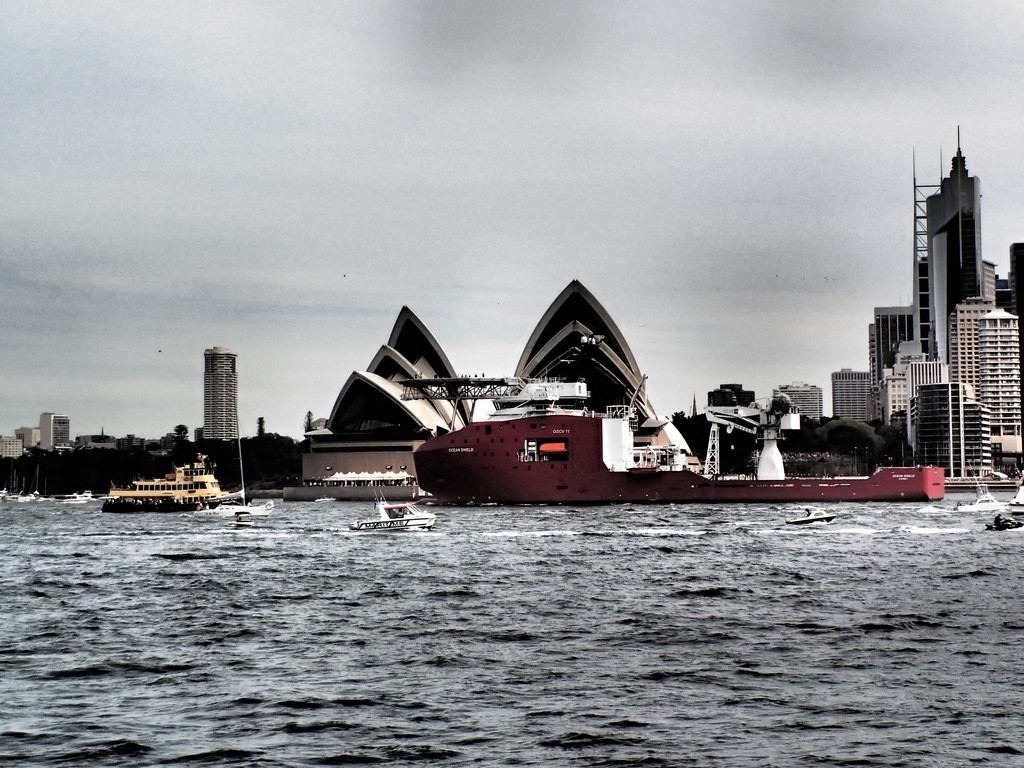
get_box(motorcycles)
[985,521,1024,532]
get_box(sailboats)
[0,466,51,503]
[193,402,274,518]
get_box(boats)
[955,459,1007,511]
[55,493,93,503]
[282,469,428,502]
[315,496,336,503]
[232,510,254,526]
[1008,465,1024,515]
[349,491,436,531]
[412,367,945,503]
[785,508,837,524]
[102,452,227,512]
